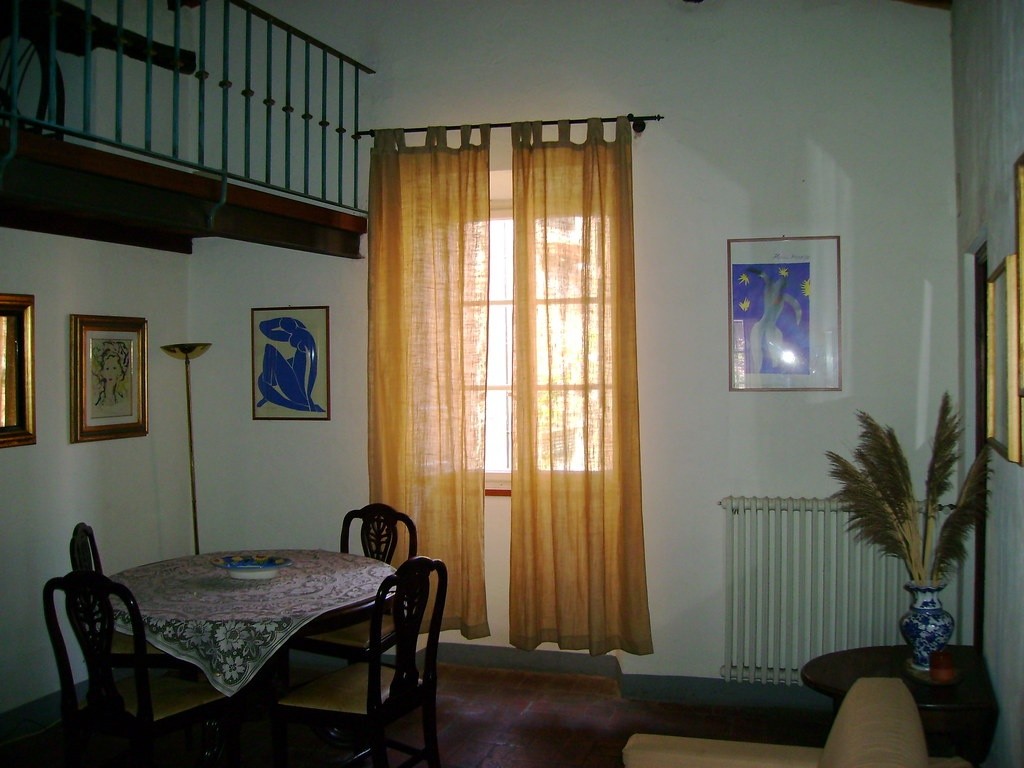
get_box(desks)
[85,550,400,700]
[801,644,999,757]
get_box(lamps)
[161,342,212,556]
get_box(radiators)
[717,495,958,688]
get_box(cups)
[930,649,954,686]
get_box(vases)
[899,583,955,670]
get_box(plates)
[208,554,294,580]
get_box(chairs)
[43,523,243,768]
[270,504,447,768]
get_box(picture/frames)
[0,293,36,449]
[727,236,842,392]
[70,314,149,444]
[985,255,1021,465]
[250,306,331,421]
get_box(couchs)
[623,673,974,768]
